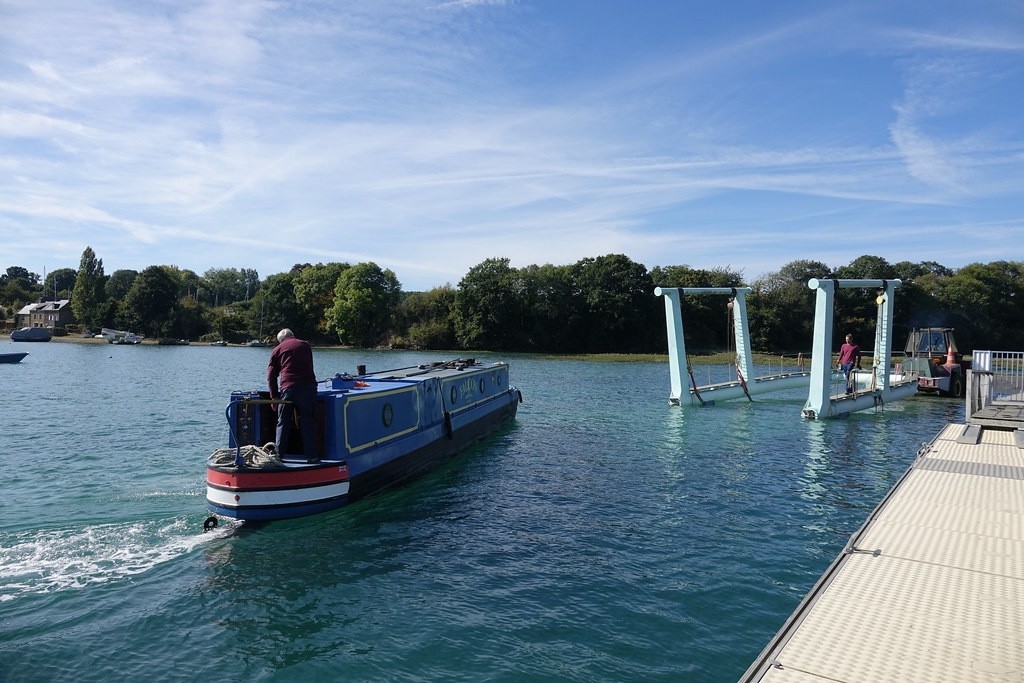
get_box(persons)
[267,328,323,463]
[837,334,862,395]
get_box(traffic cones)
[946,343,958,365]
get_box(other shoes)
[308,458,320,464]
[849,389,855,393]
[844,392,848,395]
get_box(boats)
[204,356,522,524]
[0,352,29,363]
[246,339,268,347]
[10,326,52,342]
[208,340,228,346]
